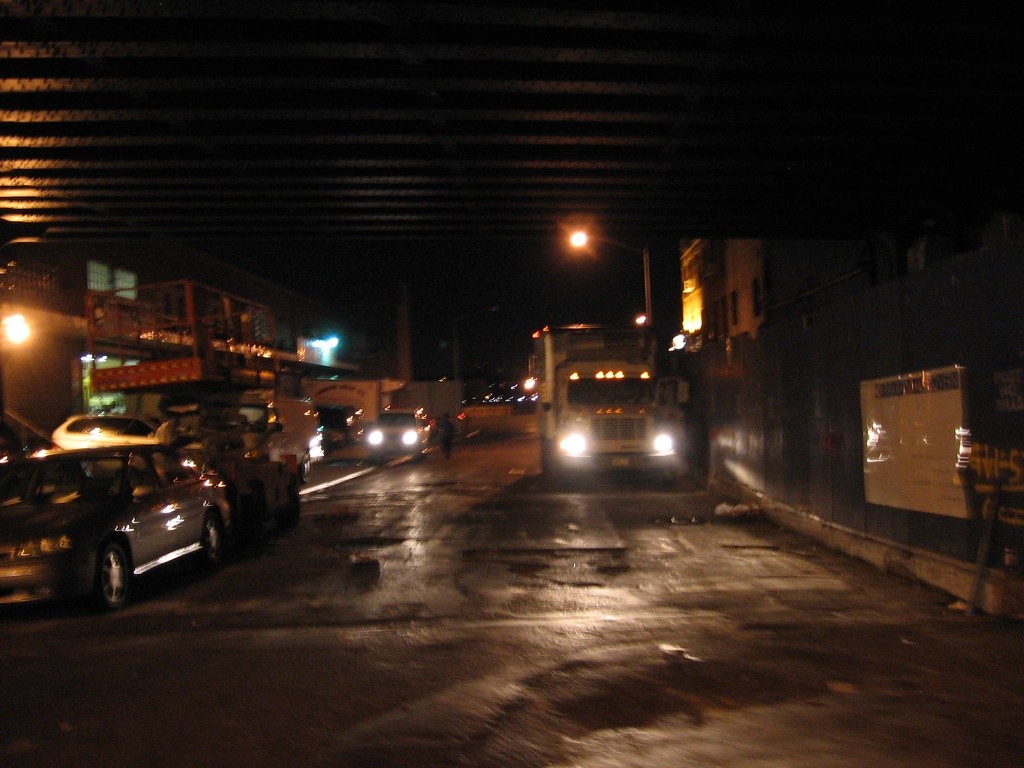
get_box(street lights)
[568,228,656,324]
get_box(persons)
[438,413,456,458]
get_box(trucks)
[528,324,693,488]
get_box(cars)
[0,411,178,453]
[364,407,436,459]
[0,443,233,614]
